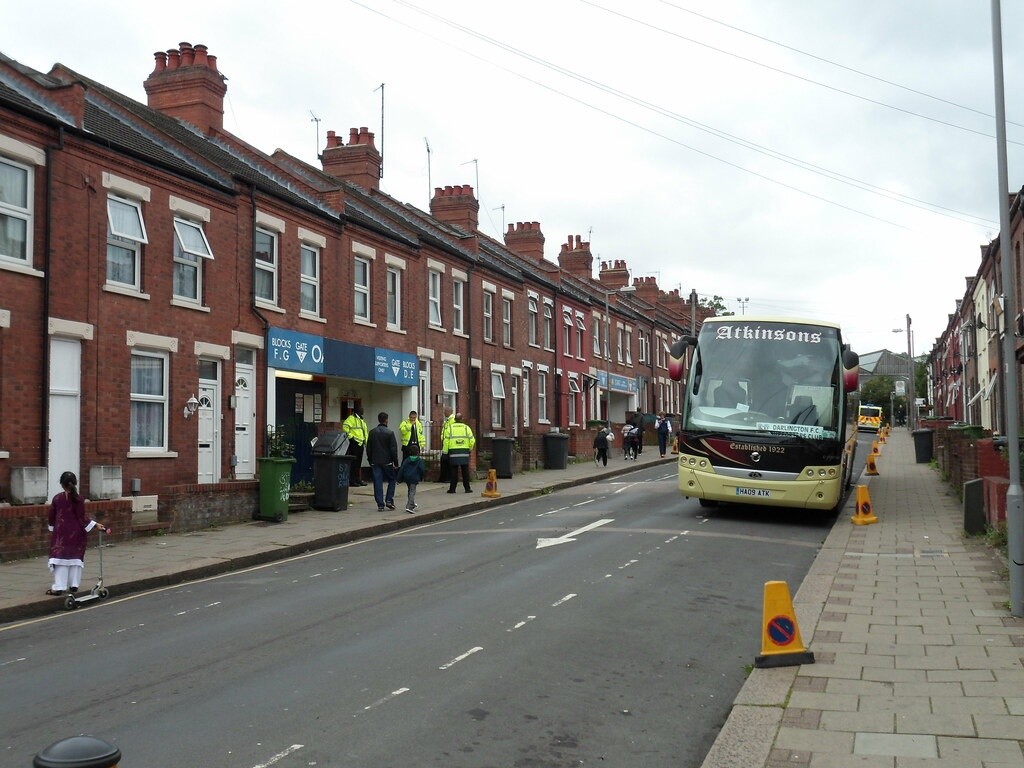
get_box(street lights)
[604,285,637,459]
[892,328,917,430]
[736,296,750,315]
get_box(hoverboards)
[64,524,112,611]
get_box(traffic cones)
[877,431,887,444]
[876,423,889,436]
[480,469,501,499]
[850,485,879,526]
[871,440,881,457]
[670,436,679,455]
[753,580,816,669]
[864,453,879,476]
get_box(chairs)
[789,396,817,426]
[714,371,746,407]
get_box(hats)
[597,426,603,432]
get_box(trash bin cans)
[491,437,516,479]
[543,432,570,470]
[254,458,297,522]
[911,428,935,463]
[311,453,358,513]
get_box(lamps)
[977,312,997,332]
[184,393,199,419]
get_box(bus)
[858,403,885,432]
[668,313,861,516]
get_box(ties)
[412,425,416,442]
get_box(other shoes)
[68,586,79,592]
[630,456,633,460]
[447,490,456,493]
[378,507,384,511]
[415,504,418,508]
[358,481,368,486]
[46,589,62,596]
[661,455,664,457]
[350,483,360,487]
[635,458,637,460]
[386,503,396,510]
[624,455,627,460]
[406,508,416,514]
[465,490,473,493]
[604,464,608,468]
[595,460,599,467]
[638,453,641,455]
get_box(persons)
[366,412,398,512]
[399,411,426,466]
[343,404,368,487]
[621,420,641,460]
[433,406,456,482]
[442,413,476,494]
[593,425,611,467]
[397,445,424,514]
[46,471,104,595]
[654,411,672,458]
[630,407,646,456]
[898,404,906,427]
